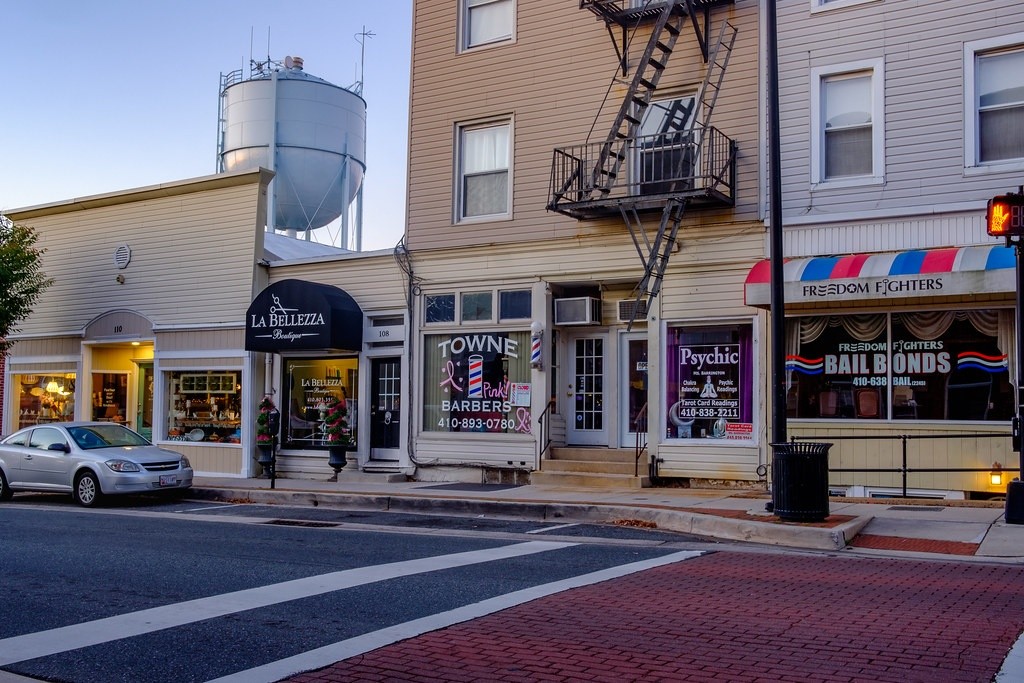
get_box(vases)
[256,444,278,479]
[324,444,350,481]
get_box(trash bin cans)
[769,441,835,523]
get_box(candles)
[210,397,215,404]
[213,405,217,411]
[186,400,190,408]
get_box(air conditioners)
[553,297,602,327]
[616,299,648,323]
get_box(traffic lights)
[987,194,1024,238]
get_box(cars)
[0,419,193,509]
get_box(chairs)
[816,385,919,420]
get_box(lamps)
[20,374,73,397]
[990,462,1002,486]
